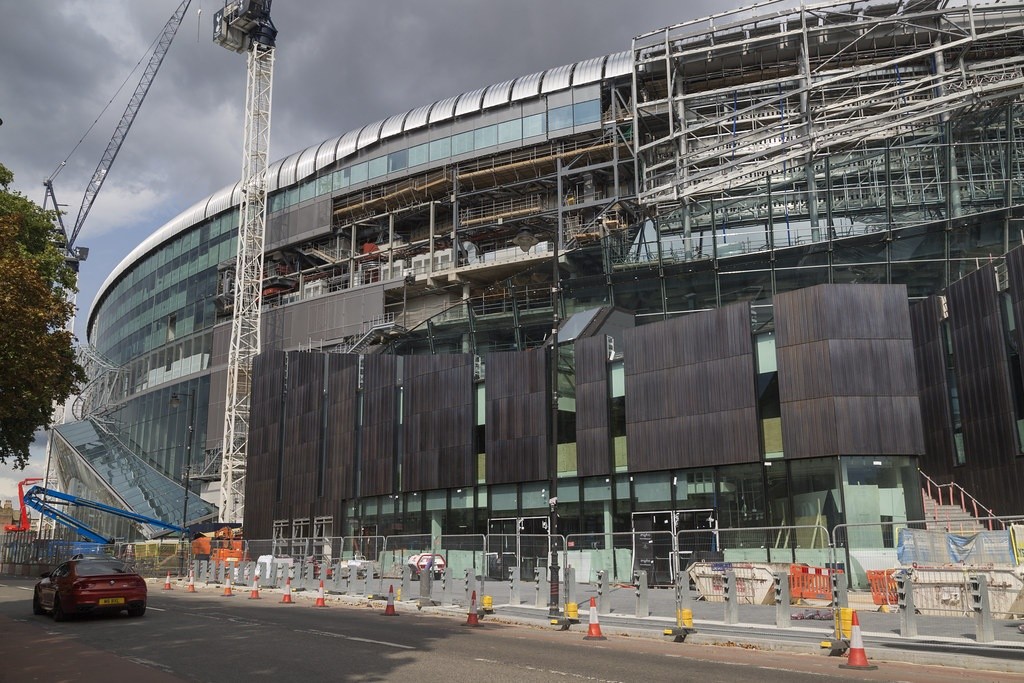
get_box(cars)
[30,556,149,619]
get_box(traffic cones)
[219,573,237,596]
[583,597,608,641]
[379,584,401,616]
[460,590,485,628]
[277,577,297,604]
[837,610,880,671]
[184,570,200,594]
[161,570,173,590]
[311,579,330,607]
[248,575,262,599]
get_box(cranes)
[37,0,279,575]
